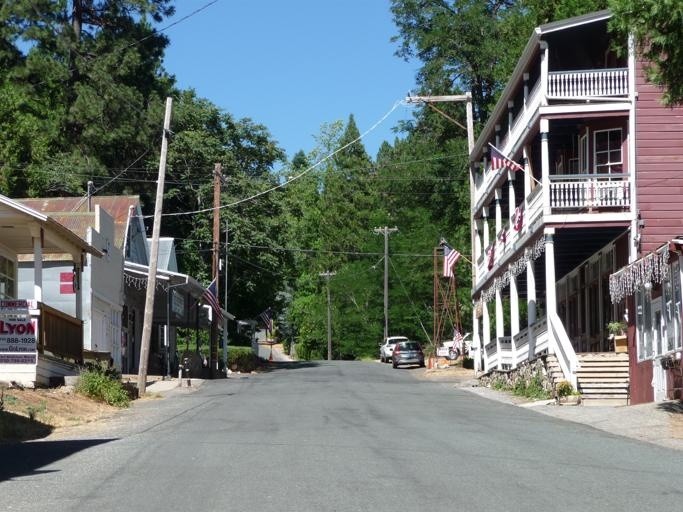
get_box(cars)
[380,336,424,368]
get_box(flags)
[202,277,223,322]
[259,310,271,328]
[490,146,522,172]
[443,242,460,278]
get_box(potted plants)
[605,320,629,353]
[556,380,581,405]
